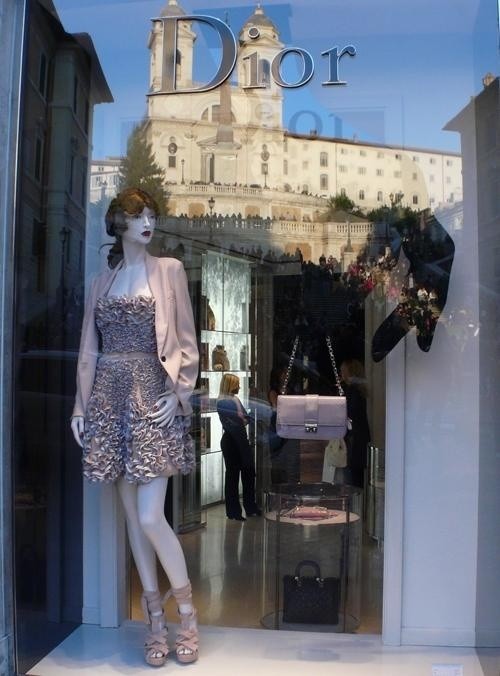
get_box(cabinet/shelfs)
[200,250,256,512]
[260,482,364,635]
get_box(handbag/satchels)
[282,560,341,627]
[275,332,350,443]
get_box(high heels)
[170,578,200,664]
[228,510,263,521]
[141,589,169,667]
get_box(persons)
[165,179,324,200]
[215,372,264,521]
[69,188,203,668]
[176,210,312,231]
[225,240,339,279]
[333,355,370,498]
[266,358,301,513]
